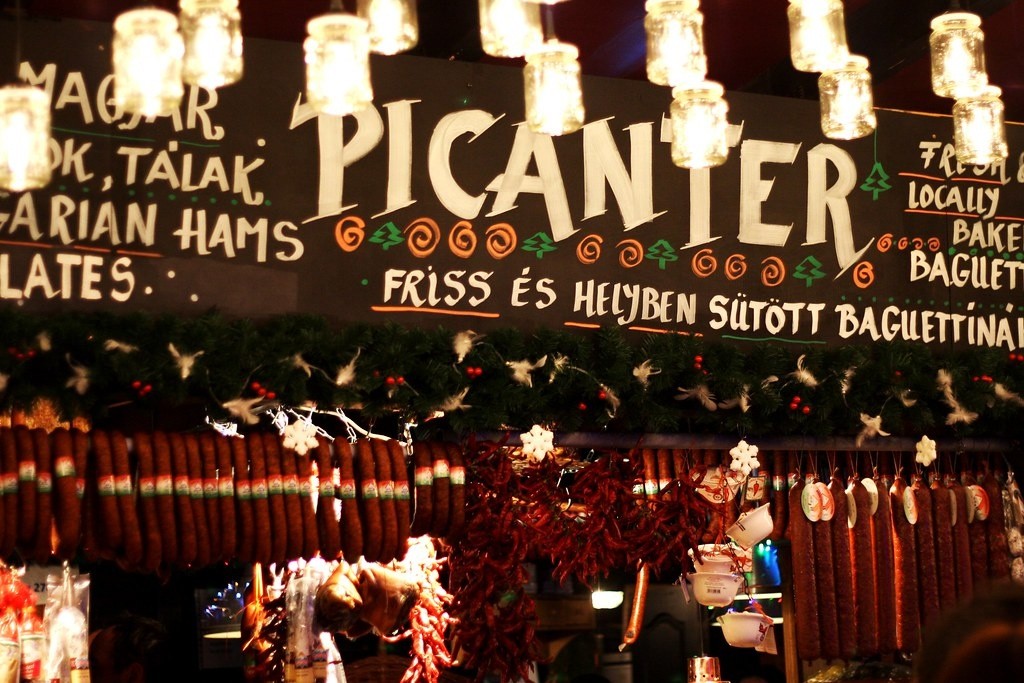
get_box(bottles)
[20,606,46,683]
[0,607,20,683]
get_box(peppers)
[399,430,712,683]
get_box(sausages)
[0,605,91,683]
[0,427,1010,658]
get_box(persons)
[908,577,1024,683]
[89,612,166,683]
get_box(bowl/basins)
[687,544,734,572]
[686,573,744,607]
[716,613,772,648]
[724,501,774,549]
